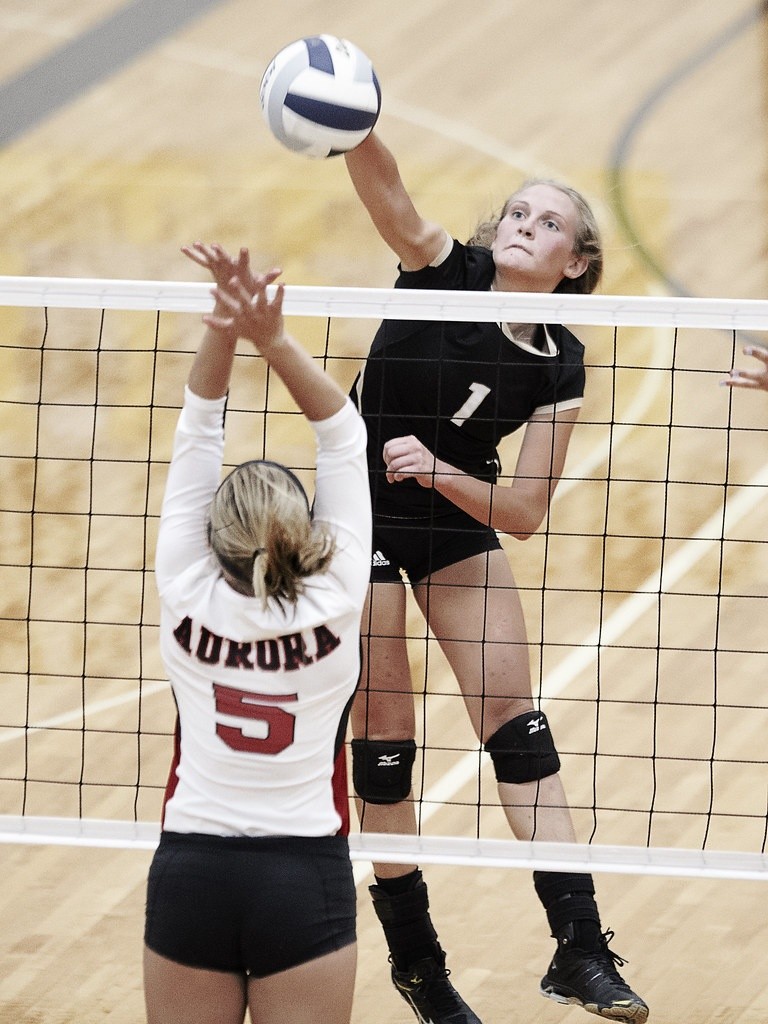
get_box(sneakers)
[540,922,650,1024]
[387,951,483,1024]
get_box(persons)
[724,345,768,392]
[141,245,372,1024]
[344,131,650,1024]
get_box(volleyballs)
[259,34,382,160]
